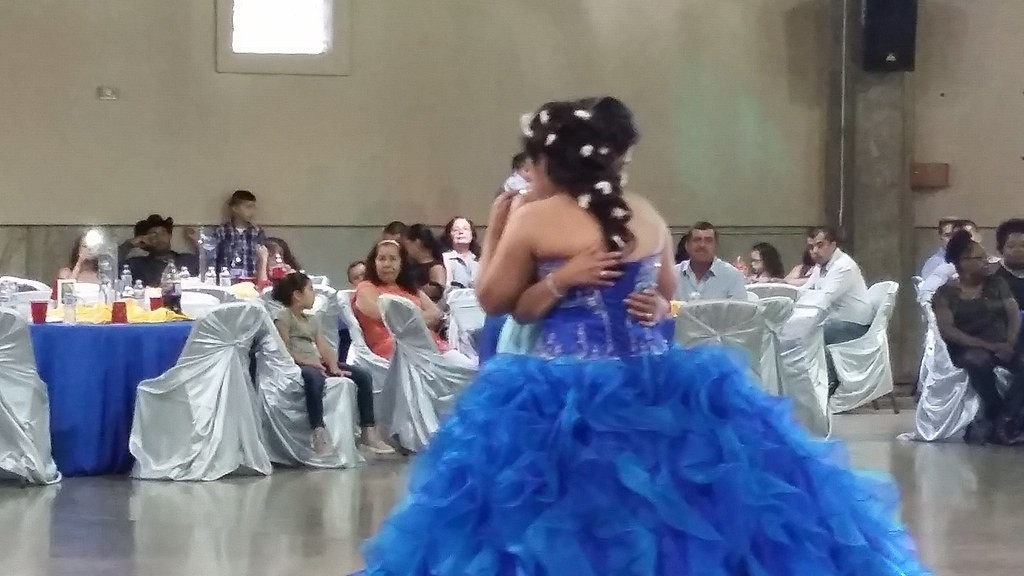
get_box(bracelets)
[546,273,563,298]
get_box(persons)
[60,190,299,290]
[272,274,395,458]
[921,217,1024,445]
[347,94,930,576]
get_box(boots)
[359,426,396,454]
[311,426,333,457]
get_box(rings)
[646,313,651,319]
[600,272,607,278]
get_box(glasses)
[958,253,988,260]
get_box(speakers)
[863,0,918,71]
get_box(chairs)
[0,256,1010,485]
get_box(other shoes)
[829,379,839,398]
[986,404,1017,446]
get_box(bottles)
[120,264,132,288]
[205,266,217,285]
[132,279,145,300]
[161,259,181,314]
[178,266,191,278]
[219,266,231,286]
[63,290,77,324]
[0,280,17,309]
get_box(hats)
[134,214,174,250]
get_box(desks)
[26,317,196,479]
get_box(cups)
[150,298,162,311]
[31,301,48,325]
[112,302,127,323]
